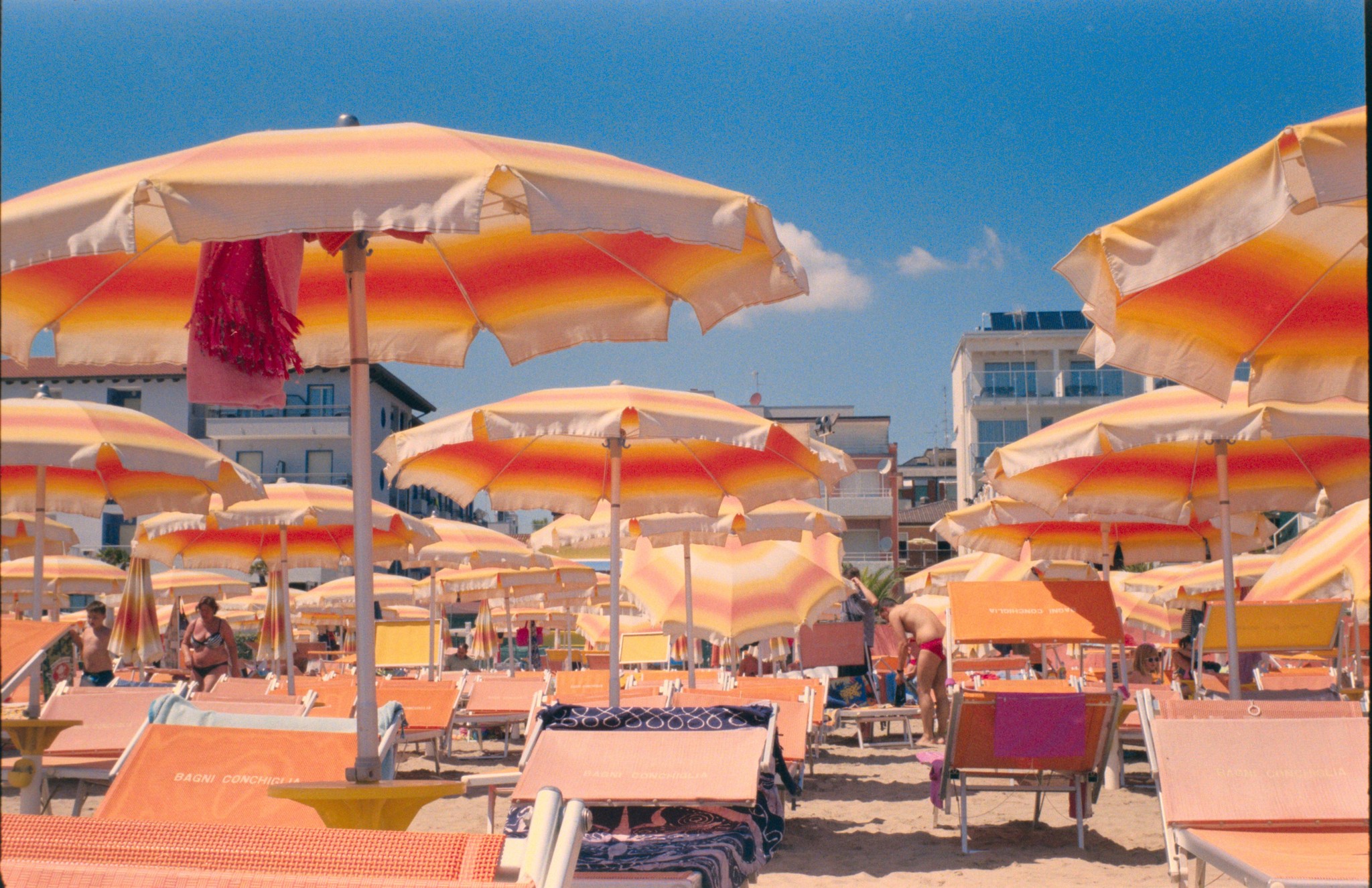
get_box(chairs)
[0,579,1370,888]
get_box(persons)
[181,596,240,691]
[69,601,114,686]
[1172,636,1197,681]
[3,510,640,671]
[844,568,878,742]
[992,641,1014,658]
[1130,643,1160,684]
[736,656,770,677]
[879,598,949,744]
[445,642,481,672]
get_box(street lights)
[813,413,835,513]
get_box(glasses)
[1145,656,1159,663]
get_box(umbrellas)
[981,379,1371,700]
[575,530,859,681]
[1243,498,1370,689]
[1,556,128,621]
[151,565,252,648]
[108,523,165,681]
[372,380,857,708]
[0,113,811,781]
[1052,101,1368,403]
[928,492,1280,694]
[0,383,269,718]
[0,512,79,557]
[256,560,297,678]
[531,497,847,691]
[903,551,1280,683]
[129,476,442,695]
